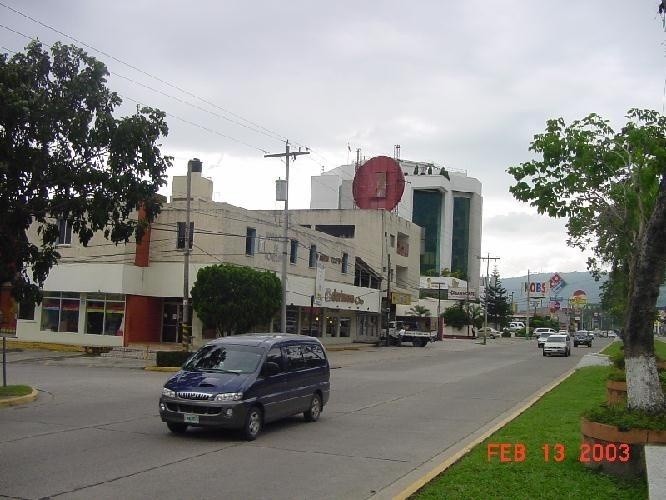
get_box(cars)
[278,320,296,332]
[479,322,617,357]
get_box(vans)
[331,319,350,336]
[159,333,330,441]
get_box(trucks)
[381,321,437,347]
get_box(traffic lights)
[550,305,560,313]
